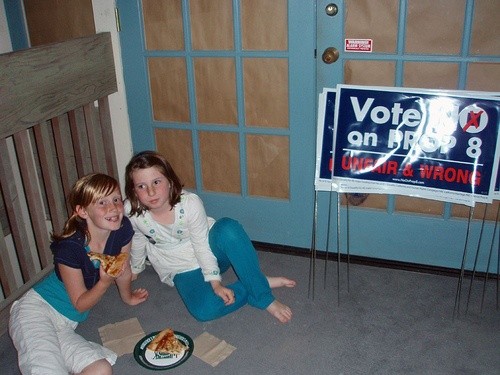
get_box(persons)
[7,172,149,375]
[113,150,297,325]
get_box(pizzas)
[146,328,188,354]
[87,251,129,277]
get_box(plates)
[133,330,194,370]
